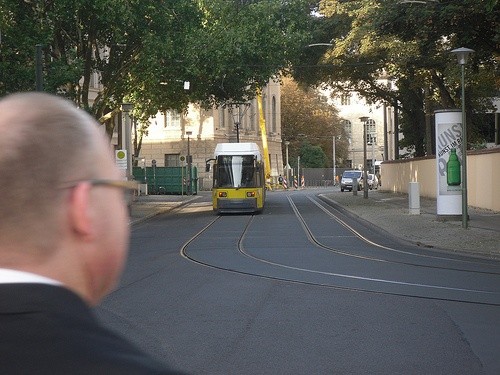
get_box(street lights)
[284,140,291,189]
[358,116,370,199]
[185,131,193,156]
[297,156,300,187]
[450,47,476,229]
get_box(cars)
[367,173,379,191]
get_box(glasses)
[57,177,148,202]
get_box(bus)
[204,142,266,215]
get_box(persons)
[266,175,272,191]
[278,175,283,185]
[0,92,184,375]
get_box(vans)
[340,169,366,191]
